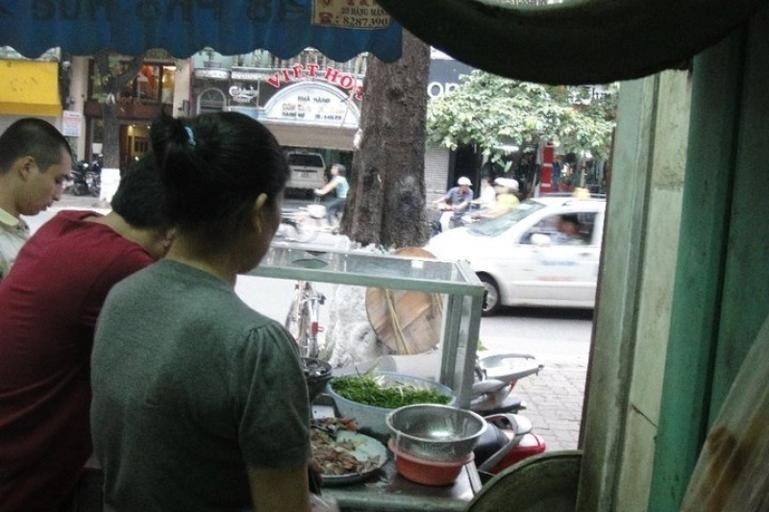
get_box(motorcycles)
[72,153,102,196]
[429,202,478,237]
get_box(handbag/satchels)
[307,197,327,218]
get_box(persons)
[0,118,73,278]
[89,111,311,512]
[432,177,520,227]
[0,152,176,512]
[555,218,590,243]
[315,164,350,229]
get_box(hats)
[494,178,519,190]
[457,176,473,186]
[560,214,578,224]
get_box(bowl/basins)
[328,370,488,487]
[304,356,332,404]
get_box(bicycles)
[277,182,347,243]
[280,217,329,357]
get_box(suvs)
[285,149,327,197]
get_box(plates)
[317,430,388,486]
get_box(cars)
[423,187,606,313]
